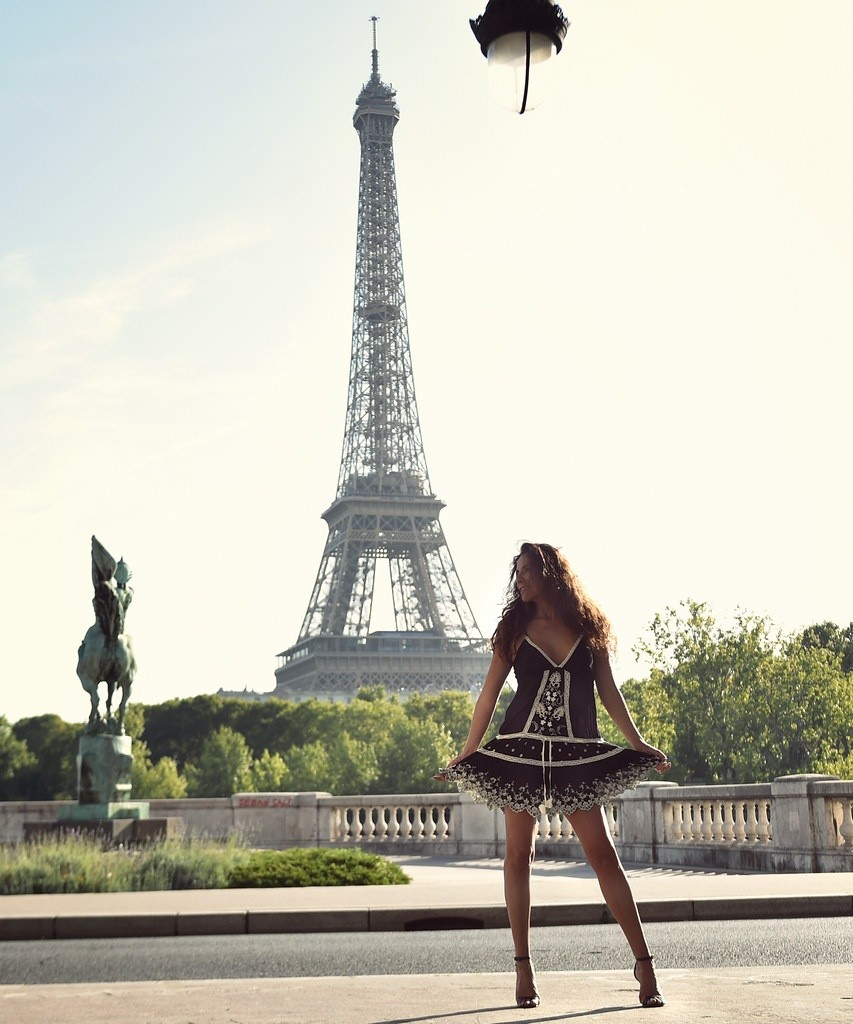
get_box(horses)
[76,568,135,735]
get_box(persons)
[433,544,672,1007]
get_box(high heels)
[634,956,665,1008]
[514,956,541,1008]
